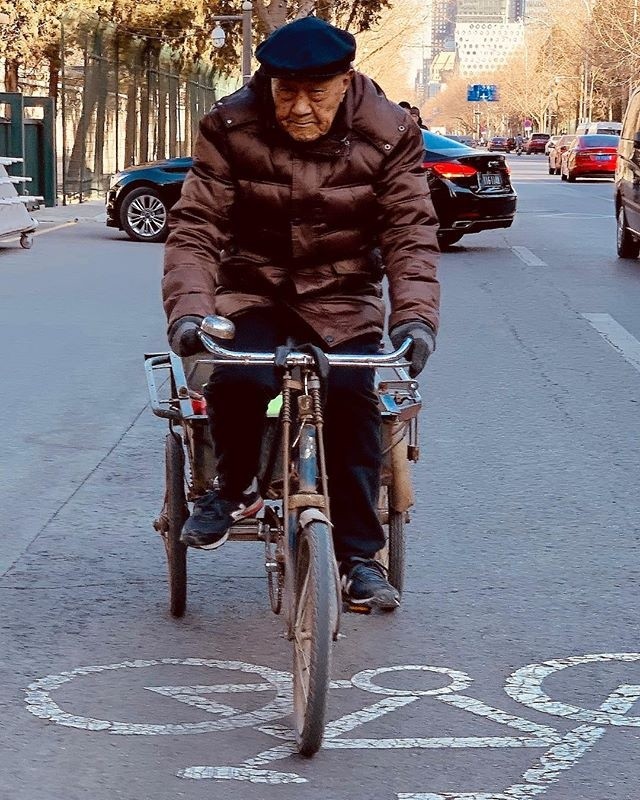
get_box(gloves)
[168,316,212,357]
[390,321,436,378]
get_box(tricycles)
[143,315,424,759]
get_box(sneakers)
[179,476,264,551]
[339,558,401,607]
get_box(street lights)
[211,0,252,86]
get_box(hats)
[255,17,357,74]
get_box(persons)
[514,134,524,151]
[161,20,441,606]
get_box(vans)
[614,85,640,259]
[576,122,623,135]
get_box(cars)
[442,135,488,148]
[544,136,562,156]
[489,137,515,153]
[560,134,619,183]
[547,135,578,175]
[526,133,550,155]
[106,129,518,251]
